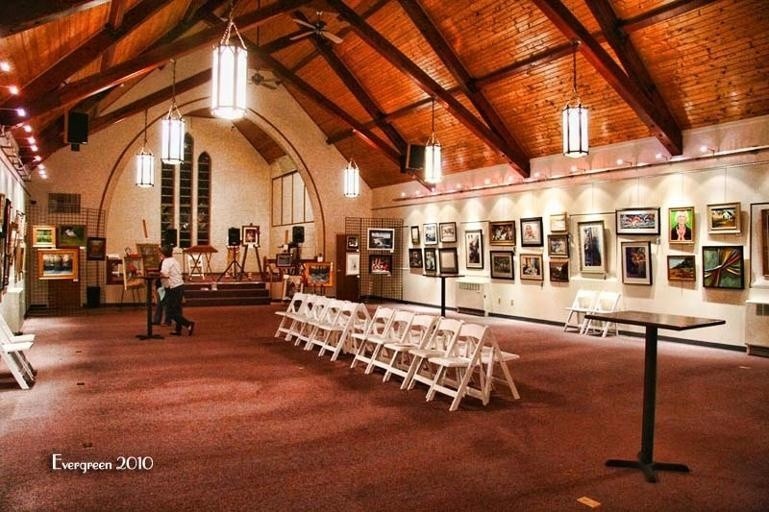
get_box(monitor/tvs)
[406,143,427,171]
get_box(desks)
[132,276,169,340]
[584,310,726,483]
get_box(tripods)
[217,247,252,282]
[288,244,306,275]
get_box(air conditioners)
[744,300,769,357]
[455,281,489,316]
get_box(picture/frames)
[242,225,260,247]
[275,253,293,267]
[0,193,106,303]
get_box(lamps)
[135,106,155,188]
[562,38,589,158]
[343,134,360,199]
[160,57,186,165]
[0,62,48,179]
[210,7,248,120]
[424,98,441,183]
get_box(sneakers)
[152,320,194,336]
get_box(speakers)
[67,111,89,145]
[292,226,305,242]
[167,228,178,247]
[228,227,240,246]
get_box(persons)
[151,247,173,328]
[671,210,692,240]
[64,225,78,240]
[621,214,655,230]
[524,224,539,242]
[493,225,512,241]
[157,242,196,337]
[426,252,435,270]
[411,251,422,267]
[246,230,255,242]
[469,241,479,263]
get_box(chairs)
[0,313,35,390]
[274,292,520,412]
[564,290,621,338]
